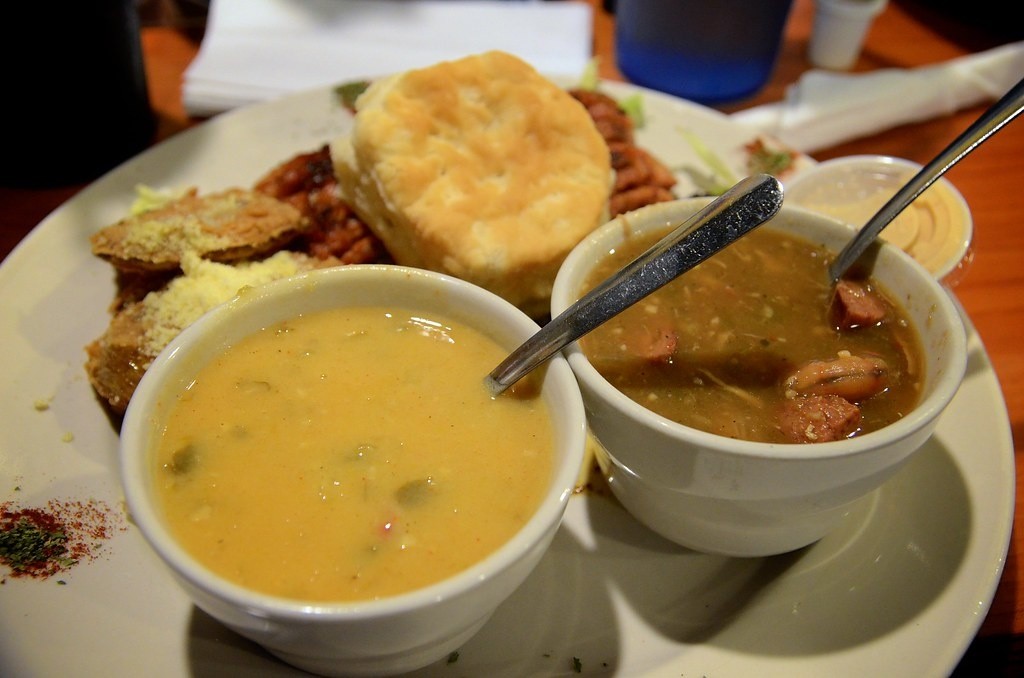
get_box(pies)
[332,50,615,320]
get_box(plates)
[0,83,1016,678]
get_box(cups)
[609,1,794,105]
[808,0,887,69]
[0,0,150,186]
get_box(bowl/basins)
[784,155,974,282]
[119,264,585,678]
[553,195,964,559]
